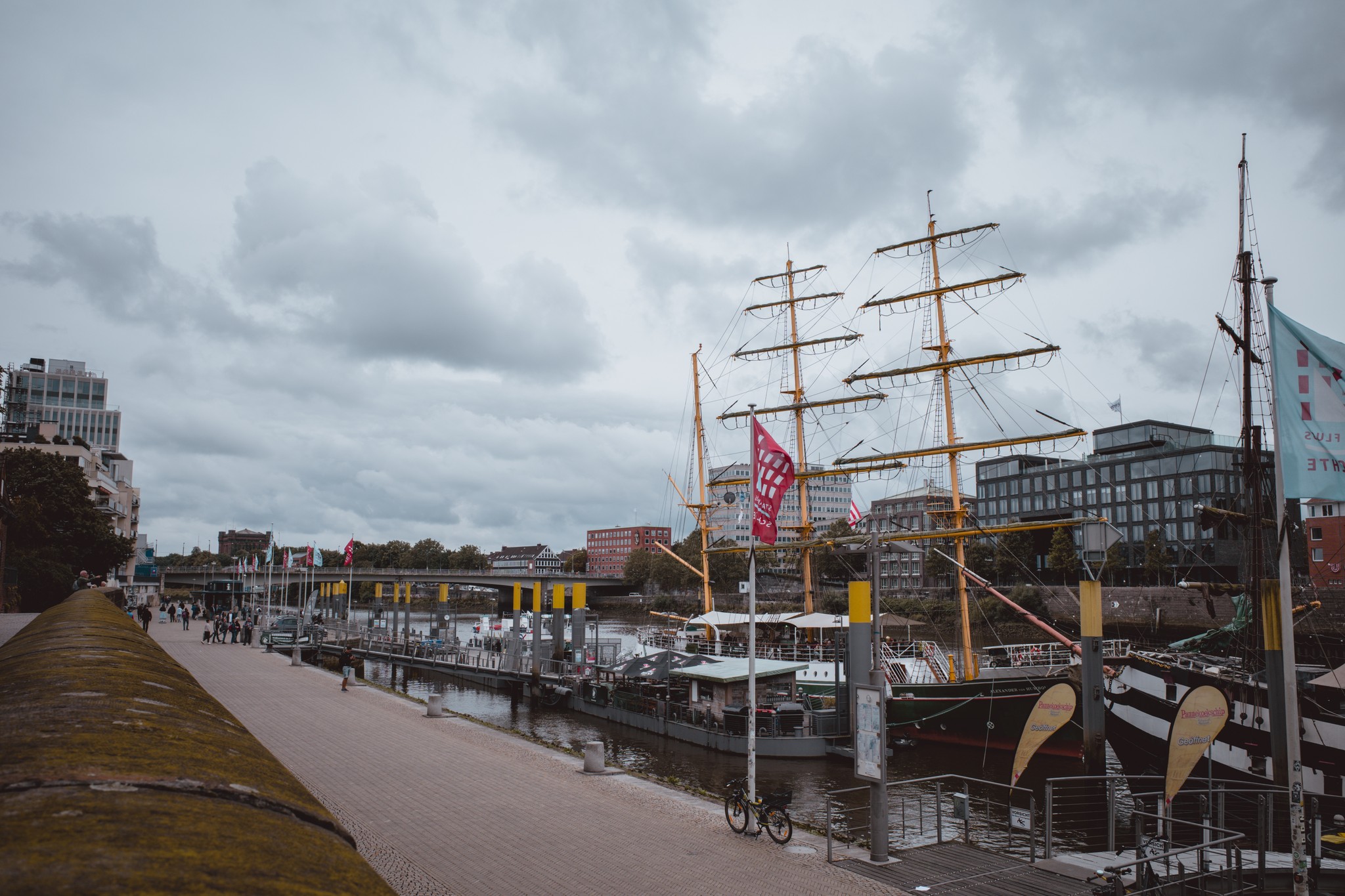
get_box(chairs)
[826,646,835,663]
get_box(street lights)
[390,546,392,568]
[520,550,523,573]
[208,540,211,565]
[259,540,262,569]
[230,542,234,566]
[483,549,486,571]
[337,546,340,570]
[523,554,544,576]
[439,548,443,571]
[282,544,285,564]
[479,546,481,574]
[488,558,509,575]
[562,551,583,572]
[426,544,429,572]
[523,547,526,570]
[155,540,157,564]
[182,543,185,569]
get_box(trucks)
[468,585,481,591]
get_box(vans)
[629,593,642,597]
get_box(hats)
[320,611,323,613]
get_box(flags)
[1269,302,1345,504]
[301,589,319,636]
[848,498,861,527]
[1008,680,1080,799]
[748,417,793,545]
[236,525,353,575]
[1164,681,1233,808]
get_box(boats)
[568,647,832,760]
[472,610,575,662]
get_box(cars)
[411,583,427,587]
[459,588,468,590]
[483,589,493,592]
[782,590,793,593]
[799,590,805,593]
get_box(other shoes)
[186,629,189,630]
[207,642,211,644]
[231,643,235,644]
[212,642,217,643]
[218,640,222,642]
[234,641,239,643]
[243,643,246,646]
[344,688,349,691]
[341,689,346,692]
[201,641,204,644]
[248,643,251,645]
[222,642,227,644]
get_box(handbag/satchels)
[219,627,224,633]
[192,613,195,618]
[198,611,200,613]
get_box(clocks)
[444,615,450,620]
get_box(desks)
[799,648,814,661]
[690,641,715,651]
[635,682,652,685]
[662,681,689,690]
[650,684,668,693]
[665,687,682,694]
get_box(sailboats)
[643,187,1121,763]
[1101,132,1345,823]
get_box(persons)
[300,608,324,625]
[412,568,415,573]
[802,636,926,662]
[182,608,190,630]
[191,566,193,571]
[482,568,486,575]
[166,566,170,571]
[159,594,201,622]
[576,572,579,576]
[275,608,281,614]
[77,570,107,591]
[341,646,355,692]
[201,603,261,646]
[126,604,152,633]
[720,633,732,647]
[492,639,501,656]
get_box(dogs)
[174,618,178,622]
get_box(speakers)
[708,580,715,586]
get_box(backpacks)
[257,608,261,612]
[180,603,184,609]
[182,612,187,619]
[248,608,251,612]
[228,625,233,632]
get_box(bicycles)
[724,776,793,844]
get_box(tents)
[599,650,721,697]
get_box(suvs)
[269,615,329,640]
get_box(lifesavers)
[926,645,933,656]
[1012,650,1022,661]
[1031,647,1040,660]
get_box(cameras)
[353,656,354,657]
